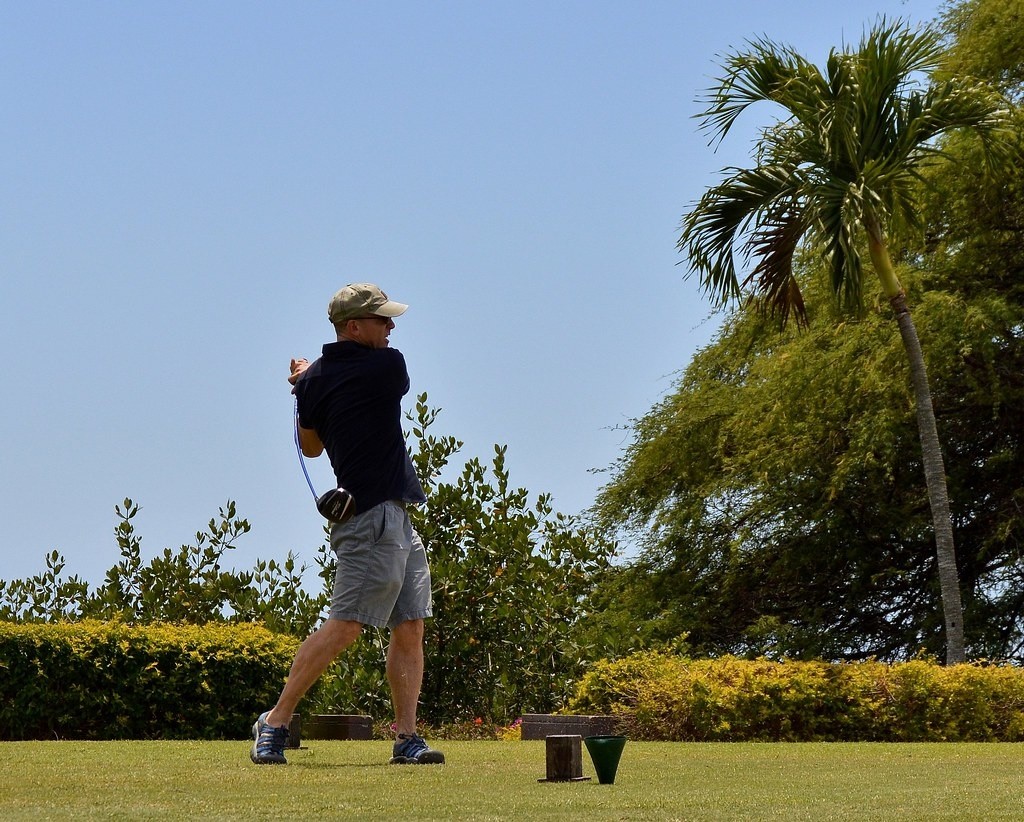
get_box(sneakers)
[386,732,444,764]
[251,712,290,764]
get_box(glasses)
[346,314,391,327]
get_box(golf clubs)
[294,393,356,525]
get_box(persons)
[250,282,445,764]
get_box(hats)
[328,282,409,323]
